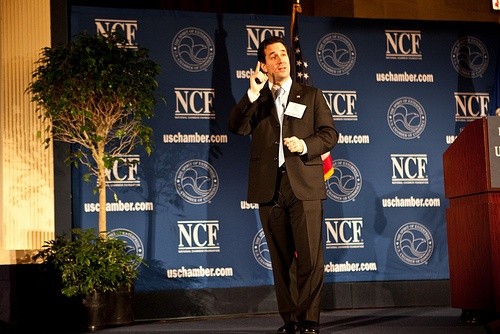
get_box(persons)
[230,36,339,334]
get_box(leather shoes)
[300,323,319,334]
[277,324,299,334]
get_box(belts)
[277,167,286,173]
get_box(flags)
[290,4,334,182]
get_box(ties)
[275,88,286,168]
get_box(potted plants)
[23,30,168,324]
[34,228,150,331]
